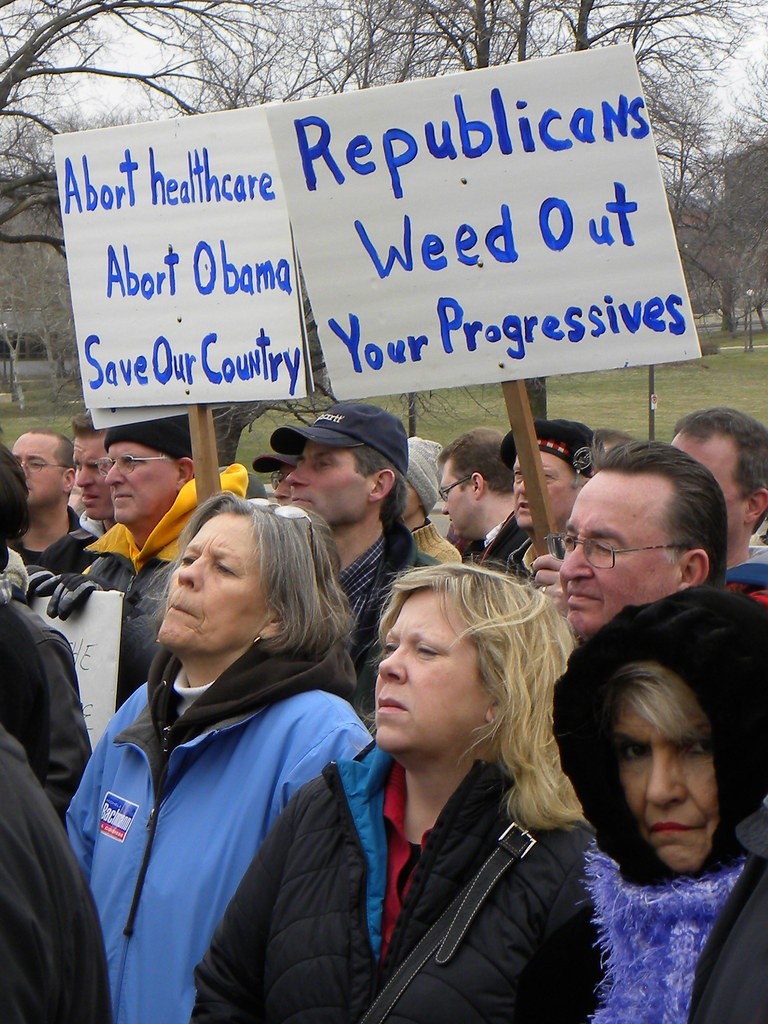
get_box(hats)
[402,436,444,517]
[102,414,193,463]
[269,402,408,477]
[500,418,597,480]
[252,453,304,472]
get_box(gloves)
[34,572,139,620]
[14,564,57,605]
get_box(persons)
[193,566,597,1024]
[67,491,374,1024]
[0,402,768,1024]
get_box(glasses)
[271,470,293,490]
[246,498,315,564]
[95,454,172,477]
[543,529,688,568]
[438,474,490,502]
[19,460,77,476]
